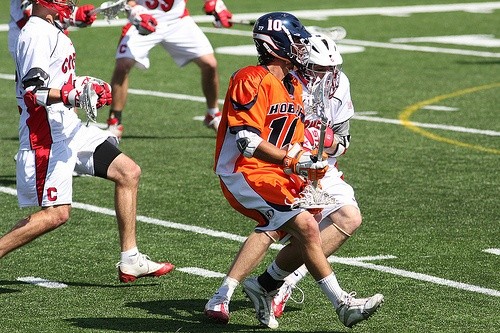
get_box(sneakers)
[204,113,221,131]
[205,296,230,322]
[115,254,175,283]
[335,292,384,328]
[271,281,305,318]
[242,276,279,329]
[107,117,121,141]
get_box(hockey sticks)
[226,18,346,41]
[294,184,343,209]
[78,82,100,119]
[87,0,132,21]
[311,72,334,188]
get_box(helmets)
[300,33,343,99]
[253,12,312,71]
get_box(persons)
[214,15,387,331]
[204,24,363,319]
[0,0,175,282]
[6,0,119,172]
[97,0,236,141]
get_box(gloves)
[59,6,97,26]
[126,4,157,36]
[203,0,232,28]
[303,125,333,150]
[282,142,328,180]
[61,76,113,109]
